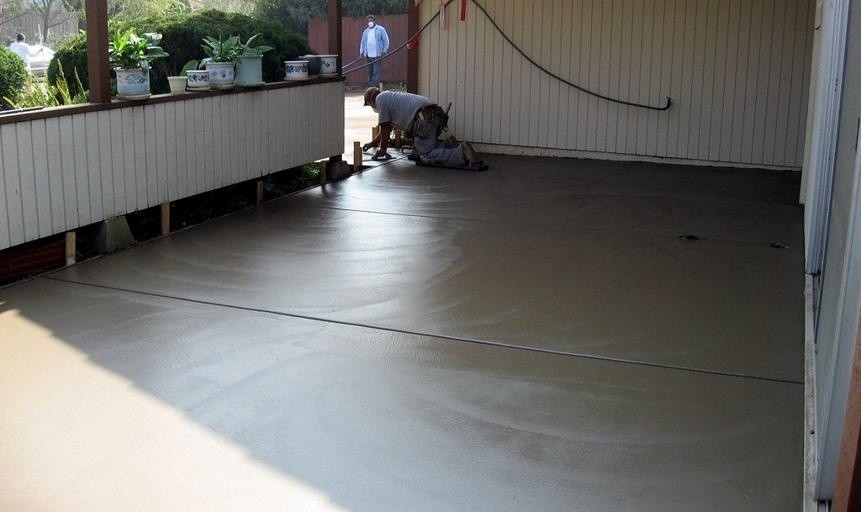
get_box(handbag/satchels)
[415,118,431,138]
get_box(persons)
[359,15,390,88]
[362,87,480,168]
[10,33,43,75]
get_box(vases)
[321,54,340,78]
[298,56,322,80]
[186,68,209,91]
[168,75,187,94]
[283,59,310,81]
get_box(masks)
[369,22,374,28]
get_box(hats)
[363,87,379,105]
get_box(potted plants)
[200,31,242,88]
[235,31,275,88]
[109,26,167,100]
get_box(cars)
[28,46,60,77]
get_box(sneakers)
[446,136,477,163]
